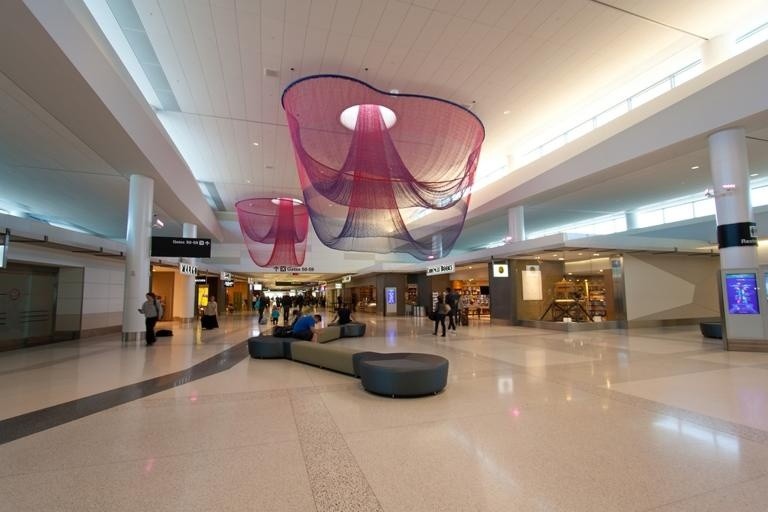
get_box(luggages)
[201,310,206,328]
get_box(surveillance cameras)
[156,218,165,228]
[722,183,738,191]
[703,186,712,197]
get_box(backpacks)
[441,303,451,315]
[449,293,459,307]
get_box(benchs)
[246,335,449,399]
[272,321,366,343]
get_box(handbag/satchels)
[254,299,260,310]
[272,324,293,338]
[429,311,440,320]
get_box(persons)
[138,292,163,346]
[432,287,465,337]
[337,293,343,308]
[291,315,322,343]
[352,293,359,312]
[204,295,219,329]
[156,296,166,321]
[331,303,357,326]
[253,290,327,327]
